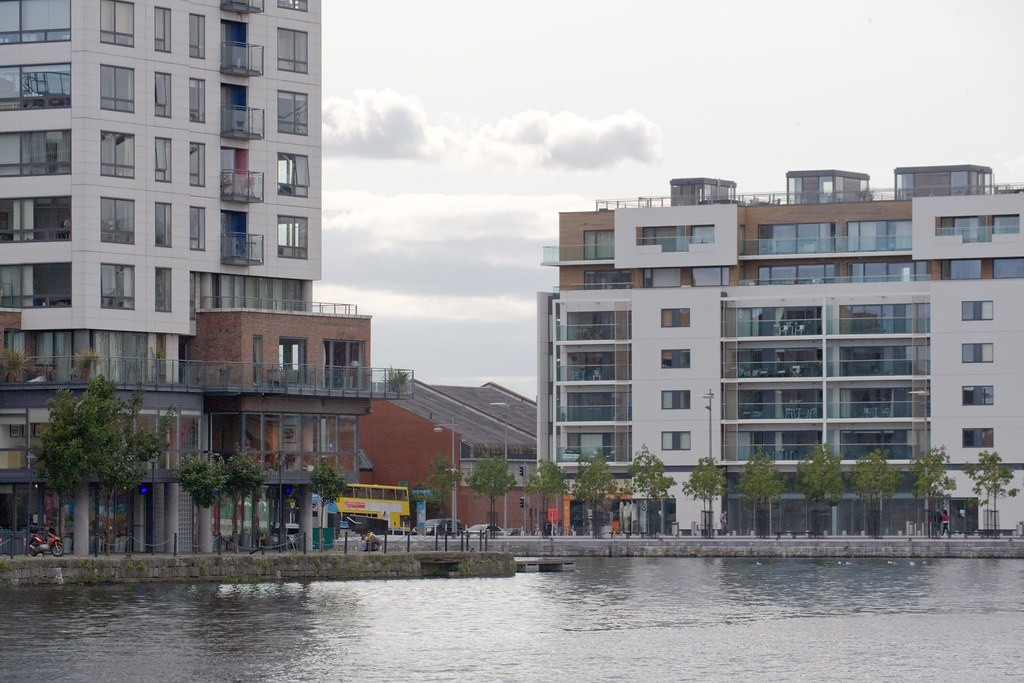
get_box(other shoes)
[941,534,943,536]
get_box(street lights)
[704,388,720,542]
[489,401,510,538]
[433,417,459,540]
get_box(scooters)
[30,526,64,557]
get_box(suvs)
[412,519,463,537]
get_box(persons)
[720,510,727,535]
[360,531,383,552]
[542,521,552,536]
[611,514,624,539]
[933,508,950,536]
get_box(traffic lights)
[520,497,526,510]
[519,462,525,477]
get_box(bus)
[339,482,411,534]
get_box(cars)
[464,522,504,538]
[505,528,521,537]
